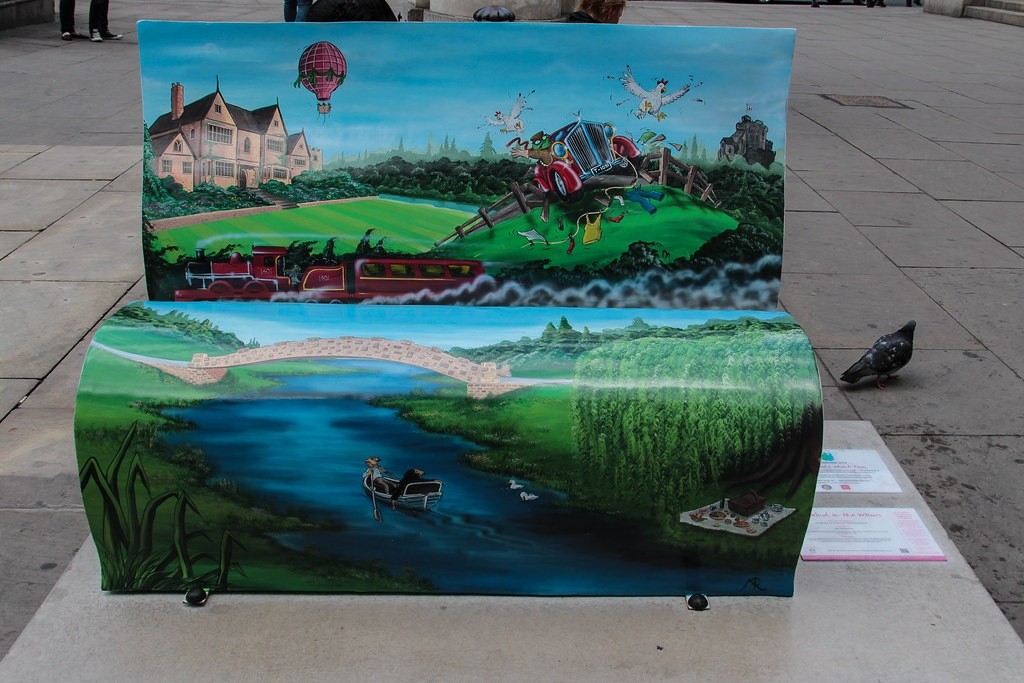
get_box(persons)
[89,0,124,41]
[283,0,313,23]
[59,0,82,41]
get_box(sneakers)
[90,30,124,41]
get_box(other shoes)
[867,1,874,7]
[61,33,89,41]
[877,2,886,7]
[906,0,912,6]
[913,0,922,6]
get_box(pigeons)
[840,321,917,391]
